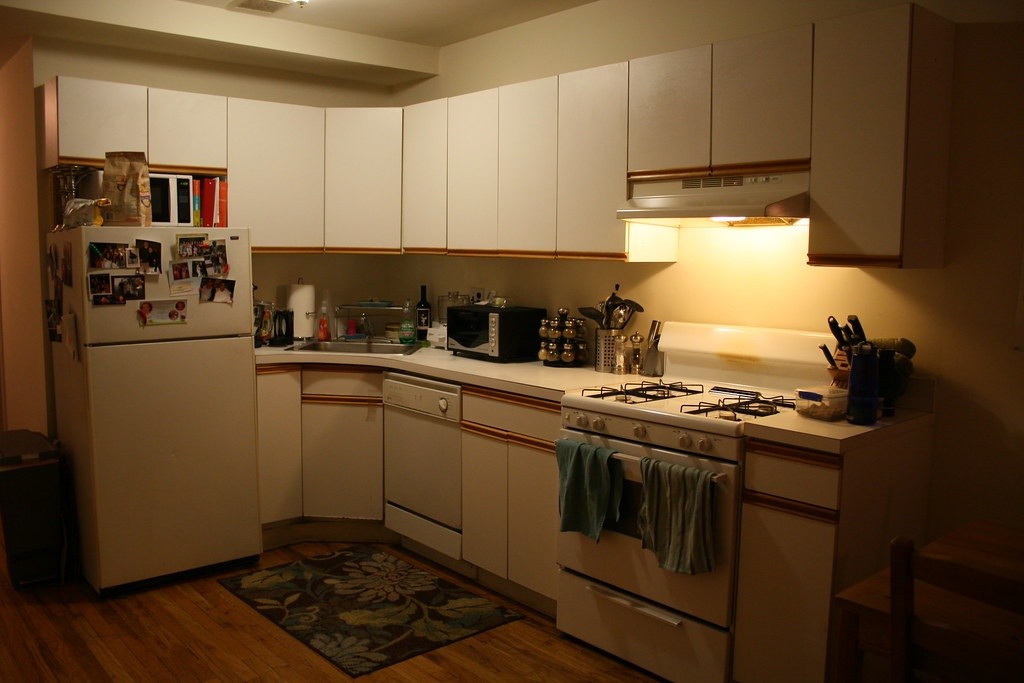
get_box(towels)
[635,456,720,576]
[555,439,624,545]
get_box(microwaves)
[445,304,548,364]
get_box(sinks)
[284,339,424,356]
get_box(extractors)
[615,161,811,230]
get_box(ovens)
[551,407,746,683]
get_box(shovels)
[578,307,605,329]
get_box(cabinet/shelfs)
[44,0,951,268]
[256,363,402,551]
[462,383,562,619]
[729,424,936,683]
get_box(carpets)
[216,544,524,678]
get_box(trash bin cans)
[0,428,71,590]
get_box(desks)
[834,520,1024,683]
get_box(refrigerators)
[46,224,264,593]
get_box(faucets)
[359,311,378,337]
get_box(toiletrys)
[398,297,416,344]
[318,307,331,341]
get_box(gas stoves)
[560,378,797,438]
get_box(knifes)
[818,314,868,369]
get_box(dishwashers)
[382,371,463,561]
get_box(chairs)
[887,535,1024,683]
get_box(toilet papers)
[286,284,316,338]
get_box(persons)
[200,279,231,303]
[119,279,139,296]
[139,242,161,272]
[197,263,201,277]
[179,240,203,257]
[210,250,225,274]
[102,251,124,269]
[173,264,188,280]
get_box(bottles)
[612,333,628,375]
[630,332,645,374]
[398,298,417,346]
[414,285,433,341]
[317,306,332,342]
